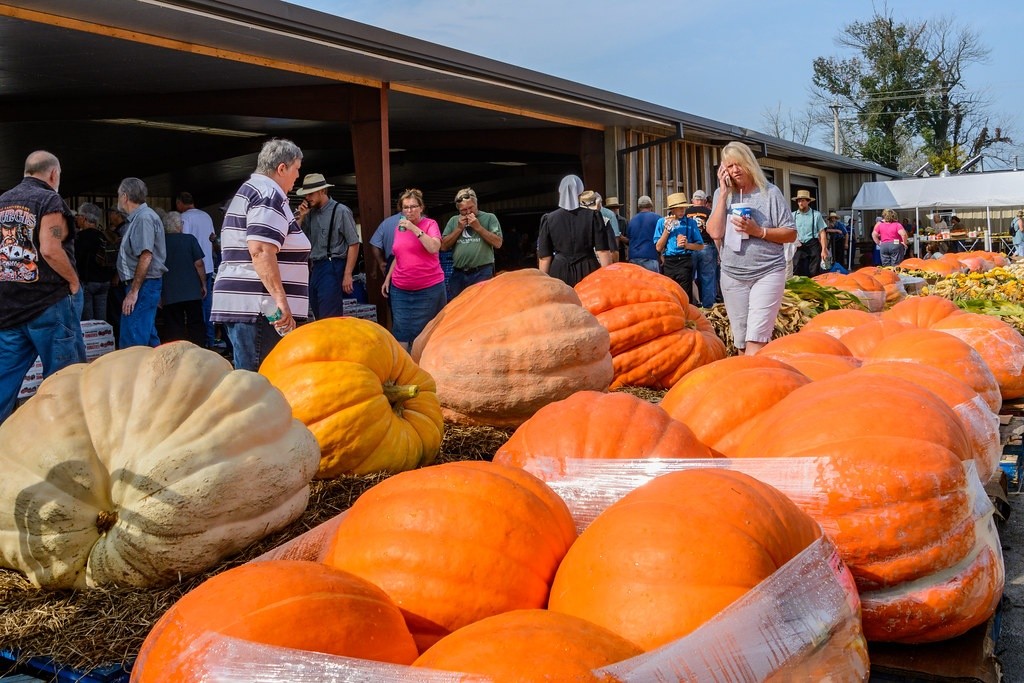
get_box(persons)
[0,141,503,430]
[536,140,966,356]
[1011,210,1024,257]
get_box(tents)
[848,171,1024,271]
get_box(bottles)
[258,295,293,339]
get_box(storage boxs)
[18,320,114,401]
[343,299,377,323]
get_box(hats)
[791,190,815,201]
[297,173,334,196]
[693,190,706,200]
[579,190,597,210]
[662,193,694,209]
[827,212,840,220]
[604,197,624,207]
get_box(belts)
[454,262,493,274]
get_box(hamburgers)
[665,215,676,219]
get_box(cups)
[731,203,752,240]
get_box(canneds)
[399,216,407,232]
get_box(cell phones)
[719,161,732,187]
[305,201,311,208]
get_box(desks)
[919,235,1016,257]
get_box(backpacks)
[1009,219,1019,237]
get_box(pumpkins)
[0,261,1024,682]
[812,251,1009,313]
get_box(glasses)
[456,194,474,203]
[180,221,184,224]
[403,204,420,209]
[74,214,88,220]
